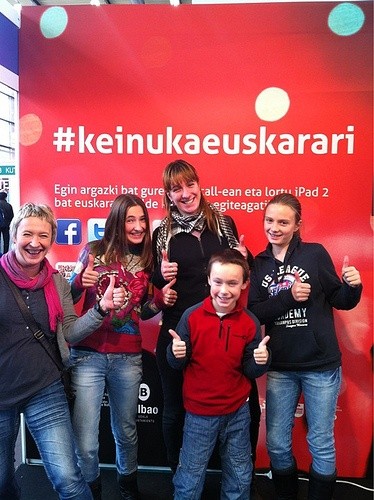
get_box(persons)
[166,249,271,500]
[245,193,363,500]
[151,160,262,500]
[71,195,177,500]
[0,202,125,500]
[0,189,14,257]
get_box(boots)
[272,455,337,500]
[87,475,102,500]
[116,470,143,500]
